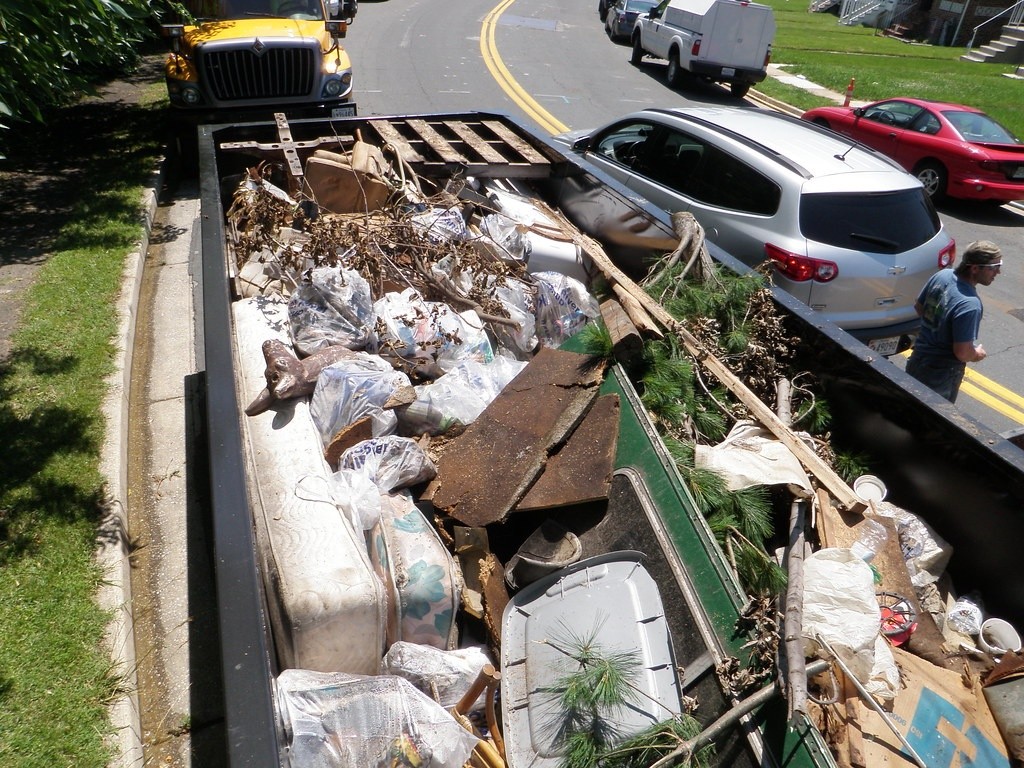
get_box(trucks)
[158,0,358,161]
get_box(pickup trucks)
[630,0,775,101]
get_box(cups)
[853,475,887,502]
[978,618,1022,655]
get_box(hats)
[962,240,1002,264]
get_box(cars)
[604,0,661,42]
[801,96,1024,209]
[598,0,616,23]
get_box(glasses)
[976,260,1002,270]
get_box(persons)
[905,241,1002,404]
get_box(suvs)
[548,104,957,358]
